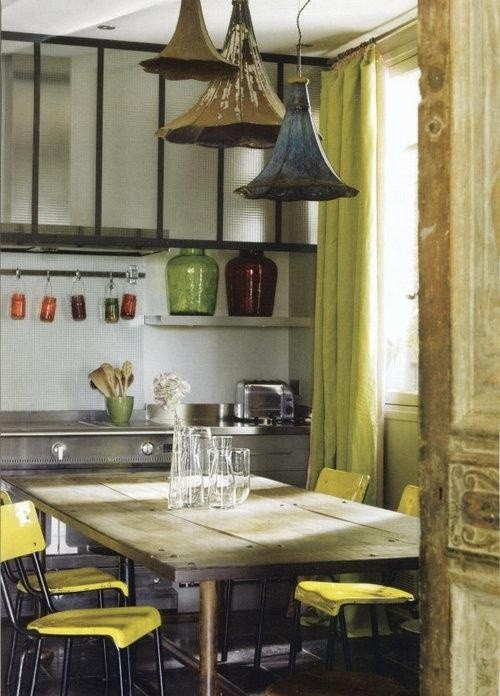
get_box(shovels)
[89,367,111,398]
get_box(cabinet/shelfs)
[232,434,310,490]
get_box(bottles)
[121,294,136,319]
[71,295,86,320]
[12,293,25,319]
[40,297,56,322]
[105,298,119,322]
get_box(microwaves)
[236,380,294,419]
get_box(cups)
[181,426,251,509]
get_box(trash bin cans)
[399,620,420,695]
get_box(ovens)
[0,433,177,614]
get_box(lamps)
[136,0,239,85]
[152,6,322,153]
[233,4,358,201]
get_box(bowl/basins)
[106,396,134,423]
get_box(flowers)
[152,371,191,417]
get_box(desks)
[0,472,421,695]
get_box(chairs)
[0,484,169,696]
[280,482,424,670]
[214,468,371,668]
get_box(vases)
[167,419,188,510]
